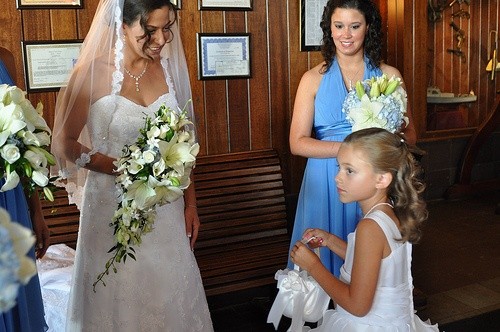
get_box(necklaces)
[124,60,149,92]
[346,75,353,91]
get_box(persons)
[286,0,417,309]
[290,128,446,332]
[49,0,215,332]
[0,59,51,332]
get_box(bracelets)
[186,204,197,209]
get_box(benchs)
[39,147,294,298]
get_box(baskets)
[267,235,331,332]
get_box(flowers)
[0,84,68,202]
[92,100,201,294]
[342,73,410,135]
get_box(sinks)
[427,93,477,101]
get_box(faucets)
[427,85,441,95]
